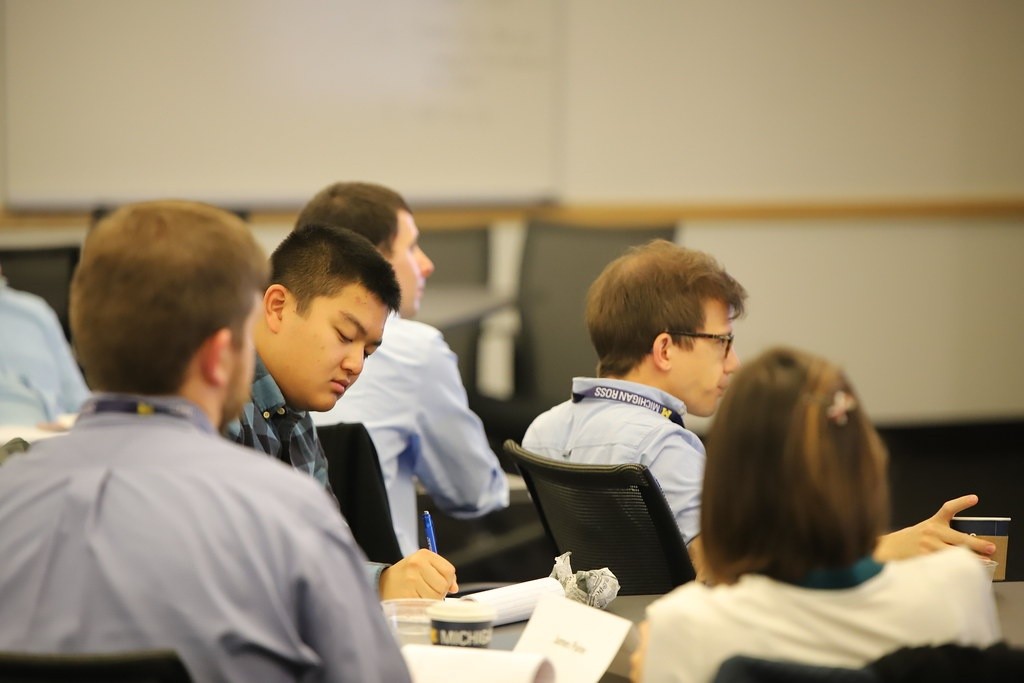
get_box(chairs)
[506,439,697,597]
[314,423,408,566]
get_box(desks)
[453,579,1024,683]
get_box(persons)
[0,275,90,431]
[221,225,459,601]
[293,180,509,558]
[643,345,1002,683]
[521,238,996,583]
[0,199,413,683]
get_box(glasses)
[666,329,736,358]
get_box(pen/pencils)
[423,510,446,602]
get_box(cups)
[950,516,1012,581]
[381,598,437,647]
[426,598,497,649]
